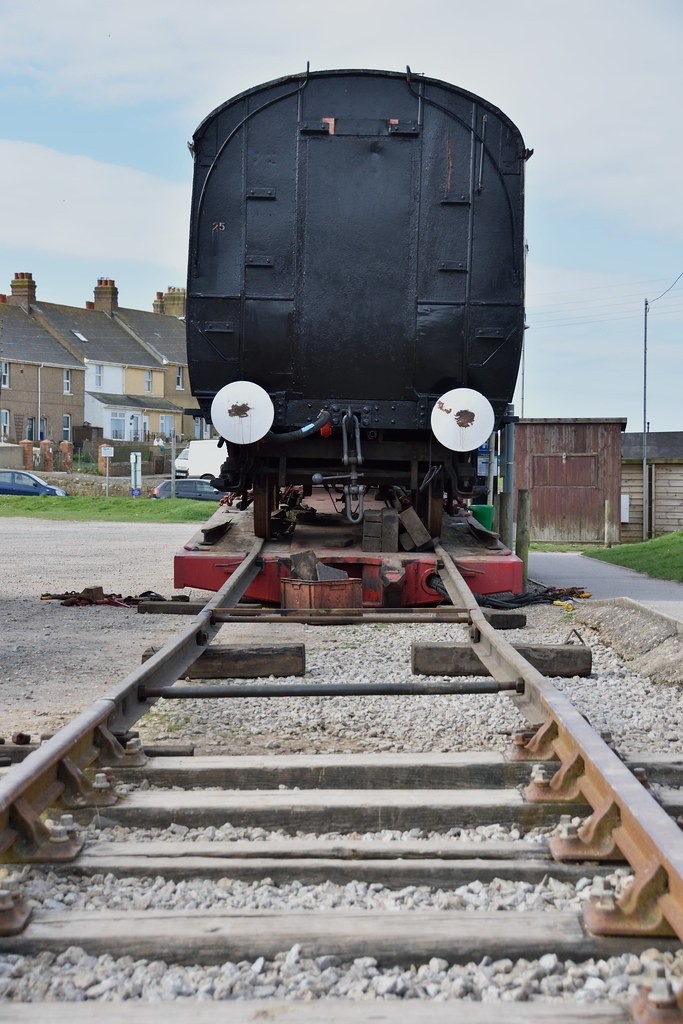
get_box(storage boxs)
[280,577,363,624]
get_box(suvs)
[149,478,231,502]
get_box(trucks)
[188,439,228,478]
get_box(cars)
[173,448,189,478]
[0,469,70,497]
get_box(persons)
[153,434,163,446]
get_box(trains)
[188,68,531,543]
